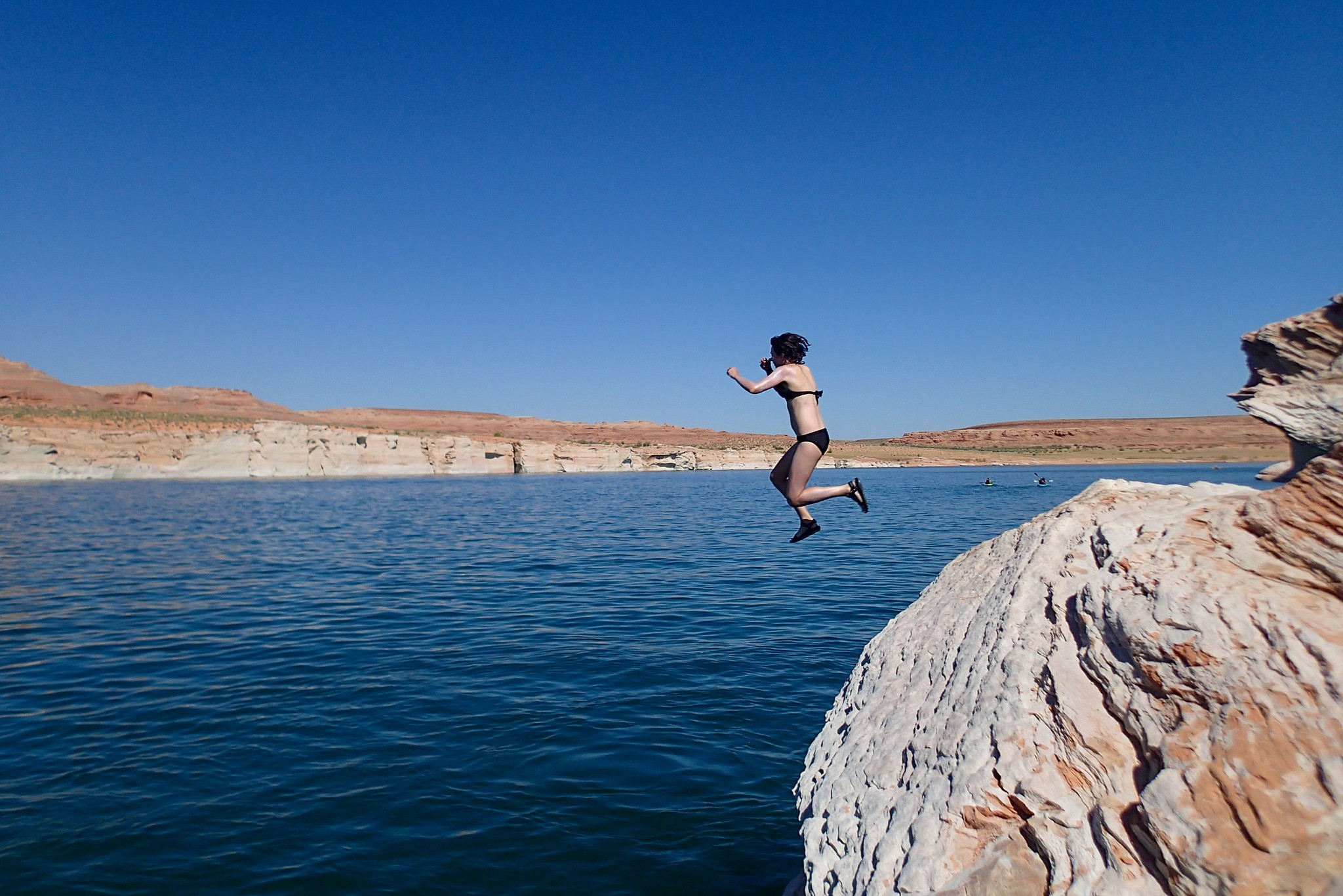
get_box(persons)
[985,478,990,484]
[1040,477,1046,483]
[726,332,868,543]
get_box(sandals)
[790,518,820,543]
[847,477,869,513]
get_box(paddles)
[1034,473,1047,485]
[1033,480,1053,482]
[979,481,996,484]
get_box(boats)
[984,480,993,486]
[1037,483,1048,487]
